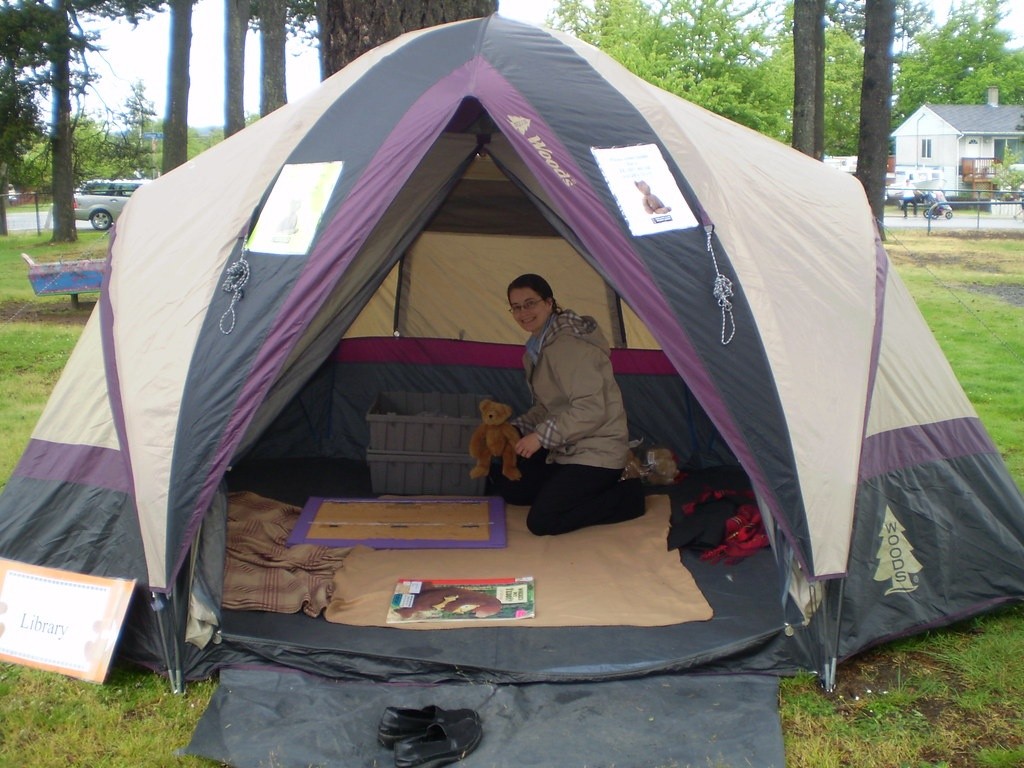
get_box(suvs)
[71,179,156,229]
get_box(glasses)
[509,299,543,315]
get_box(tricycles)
[923,196,952,220]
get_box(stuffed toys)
[470,400,523,480]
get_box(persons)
[902,179,923,218]
[488,273,679,536]
[1013,181,1024,222]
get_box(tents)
[1,13,1024,768]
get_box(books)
[386,576,536,625]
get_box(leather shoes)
[377,705,482,768]
